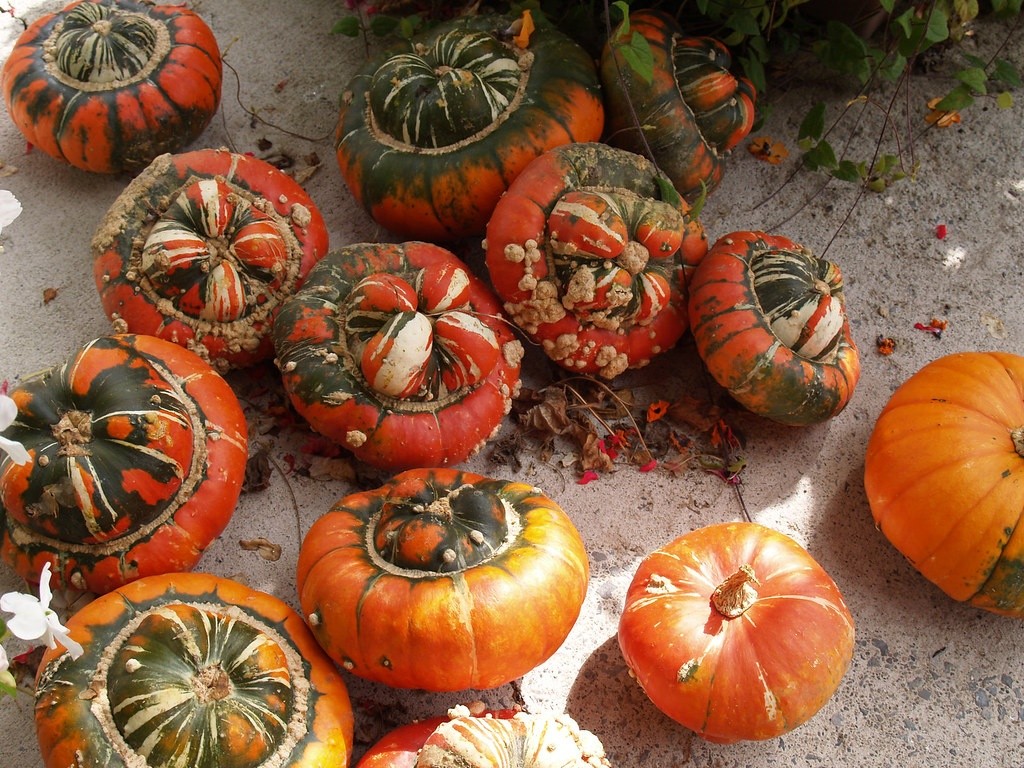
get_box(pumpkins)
[0,0,1024,768]
